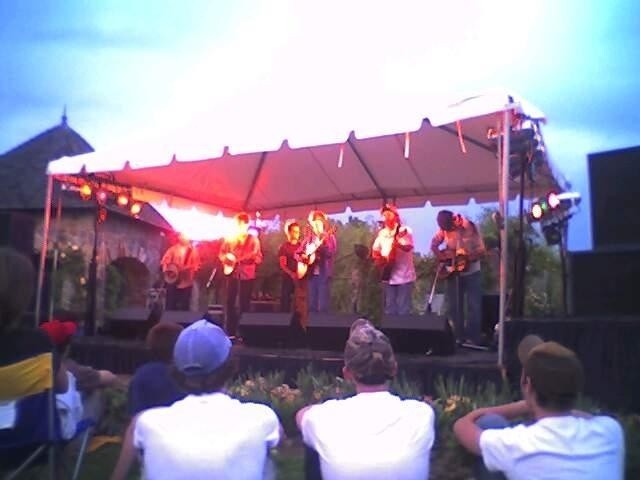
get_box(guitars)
[164,261,209,286]
[297,223,338,280]
[223,249,261,277]
[434,243,498,279]
[374,223,402,286]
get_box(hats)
[518,335,583,402]
[174,320,232,377]
[39,320,76,345]
[344,319,394,385]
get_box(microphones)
[376,220,386,229]
[205,267,217,288]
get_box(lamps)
[488,117,545,178]
[69,172,141,217]
[526,188,583,244]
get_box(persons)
[452,333,629,479]
[369,203,418,315]
[292,208,341,312]
[293,318,443,479]
[126,321,190,415]
[429,209,489,349]
[109,318,290,480]
[41,318,130,393]
[160,232,201,312]
[216,210,264,345]
[274,220,307,315]
[1,247,86,473]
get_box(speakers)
[159,310,206,333]
[238,311,303,349]
[588,147,640,247]
[306,313,362,352]
[566,249,640,321]
[107,307,155,338]
[379,314,453,352]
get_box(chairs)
[0,352,96,480]
